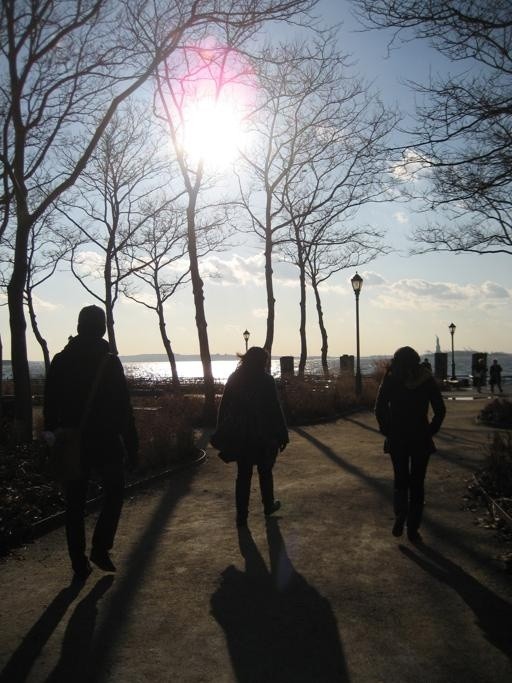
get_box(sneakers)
[88,549,116,573]
[392,513,406,539]
[409,532,424,543]
[263,499,280,516]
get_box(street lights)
[349,270,363,398]
[243,328,249,351]
[448,322,458,381]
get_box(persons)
[373,346,447,546]
[41,304,140,575]
[489,359,504,393]
[209,346,290,530]
[421,358,432,371]
[473,358,489,393]
